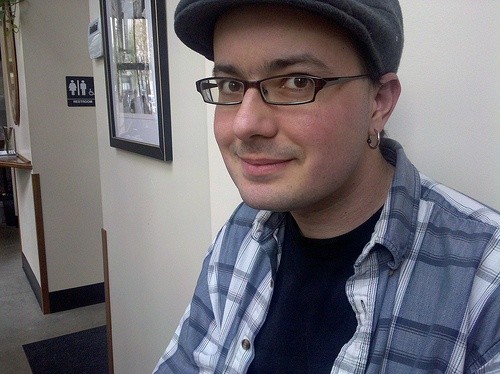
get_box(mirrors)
[1,2,20,125]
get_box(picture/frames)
[98,0,174,163]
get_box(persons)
[152,0,500,374]
[121,89,153,114]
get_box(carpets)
[21,325,109,374]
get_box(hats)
[174,0,404,77]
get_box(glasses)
[196,73,367,106]
